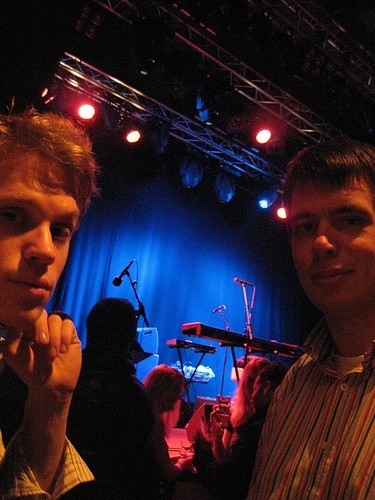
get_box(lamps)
[76,98,288,221]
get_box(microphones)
[113,259,134,286]
[212,306,223,313]
[234,278,254,286]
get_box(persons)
[56,298,179,500]
[0,107,95,500]
[143,363,194,477]
[247,139,375,500]
[208,359,291,500]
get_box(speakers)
[136,326,158,354]
[184,403,215,447]
[134,354,159,383]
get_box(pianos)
[181,322,300,358]
[166,339,217,355]
